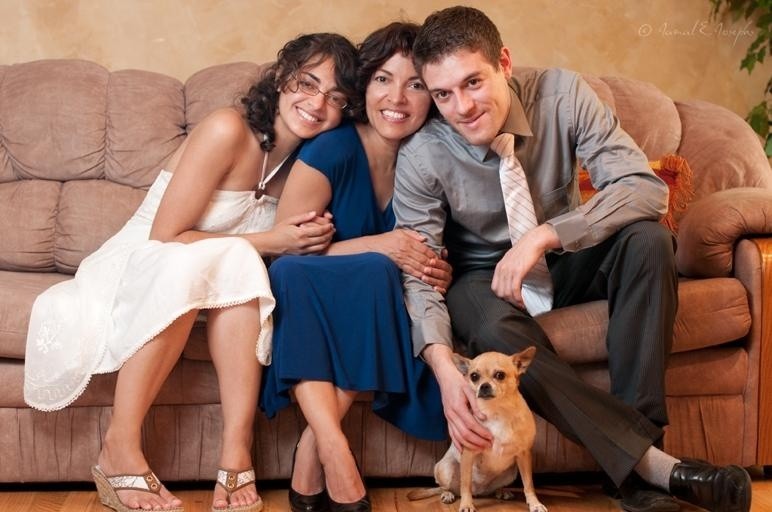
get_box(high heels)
[211,468,264,512]
[91,464,184,512]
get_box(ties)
[489,132,555,317]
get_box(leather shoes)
[602,473,680,511]
[669,458,752,511]
[289,439,371,512]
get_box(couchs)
[0,59,772,486]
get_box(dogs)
[406,344,549,511]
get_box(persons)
[390,4,754,512]
[21,31,361,512]
[257,22,456,512]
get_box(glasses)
[291,73,349,110]
